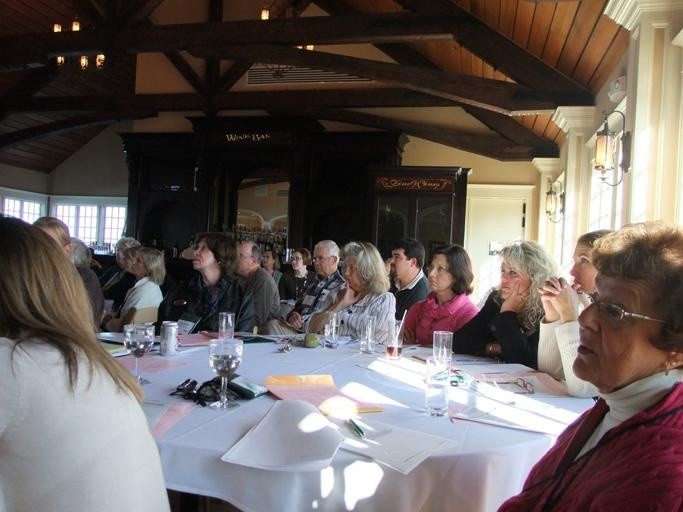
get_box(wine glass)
[122,324,155,384]
[208,338,244,411]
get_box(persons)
[404,244,479,348]
[305,242,396,335]
[385,238,431,321]
[498,219,683,512]
[32,216,166,332]
[452,241,555,369]
[0,216,170,512]
[163,239,345,334]
[538,229,612,398]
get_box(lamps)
[545,179,564,223]
[594,111,631,186]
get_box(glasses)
[495,378,535,397]
[575,288,667,324]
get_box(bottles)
[230,234,285,256]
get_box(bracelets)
[489,344,492,354]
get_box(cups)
[217,311,235,339]
[323,311,336,343]
[359,316,377,353]
[385,319,405,361]
[423,356,450,417]
[432,330,454,378]
[103,299,114,314]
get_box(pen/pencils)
[349,419,366,438]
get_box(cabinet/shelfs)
[359,163,472,277]
